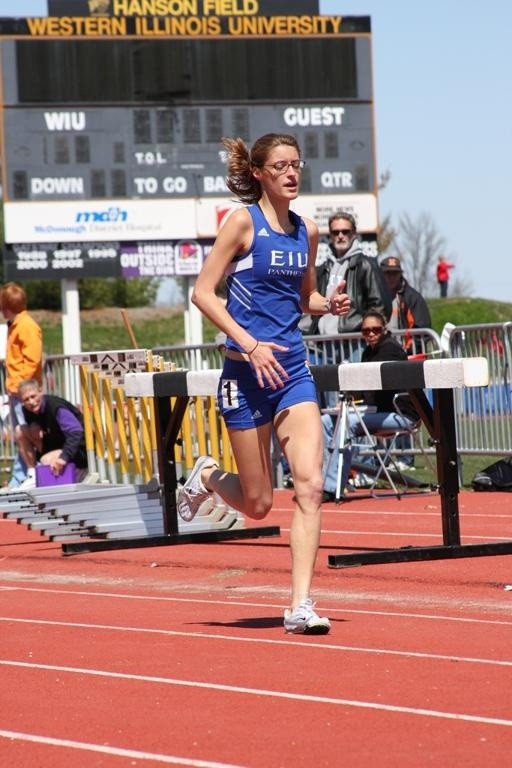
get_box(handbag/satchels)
[472,456,511,491]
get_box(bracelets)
[248,340,259,354]
[323,296,334,316]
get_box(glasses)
[361,327,382,335]
[331,229,350,236]
[265,159,305,174]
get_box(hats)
[379,257,401,272]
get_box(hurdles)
[1,348,511,567]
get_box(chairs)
[371,355,439,500]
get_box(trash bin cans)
[462,382,511,417]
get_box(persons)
[381,256,433,358]
[1,282,42,488]
[319,313,423,502]
[436,256,454,299]
[4,378,92,490]
[176,130,350,637]
[315,212,391,489]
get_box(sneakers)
[16,476,36,491]
[283,599,330,634]
[386,462,416,472]
[178,456,219,522]
[349,472,374,487]
[292,491,332,504]
